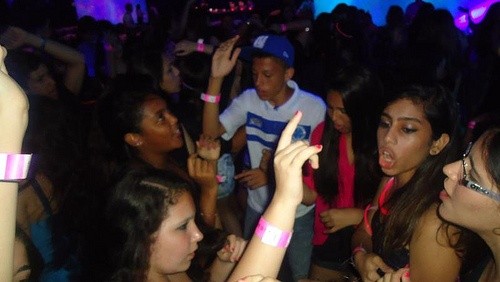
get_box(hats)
[242,34,295,68]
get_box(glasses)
[461,141,500,201]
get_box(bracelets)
[201,212,217,218]
[200,89,221,104]
[196,38,205,52]
[350,246,367,269]
[255,216,293,249]
[0,153,33,182]
[280,24,287,32]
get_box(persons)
[0,0,500,282]
